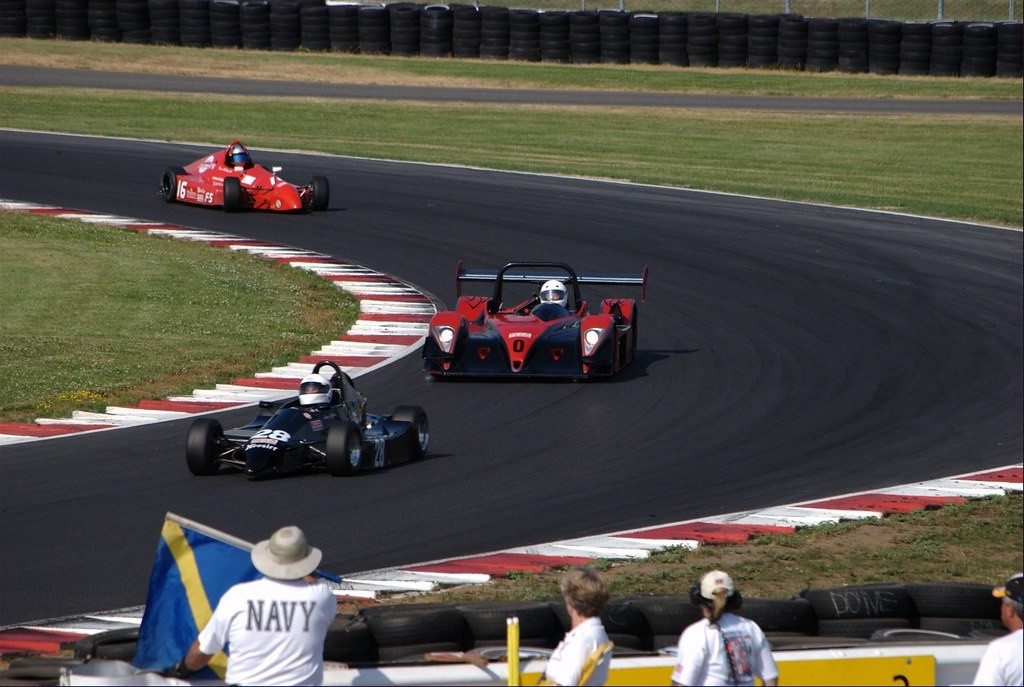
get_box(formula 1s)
[158,138,331,214]
[182,359,430,481]
[420,259,652,381]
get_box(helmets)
[228,144,249,166]
[297,374,332,405]
[538,280,568,308]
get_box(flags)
[133,518,342,682]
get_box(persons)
[671,569,779,686]
[539,279,568,308]
[544,566,612,686]
[974,573,1023,686]
[179,526,338,686]
[297,373,333,413]
[229,144,249,166]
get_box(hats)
[991,573,1024,603]
[699,570,735,600]
[250,525,323,580]
